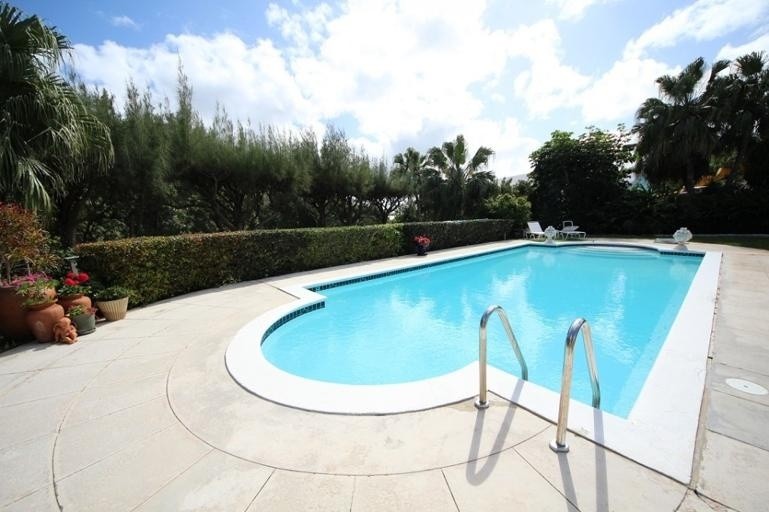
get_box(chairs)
[525,220,588,240]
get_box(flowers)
[0,271,96,337]
[414,235,432,247]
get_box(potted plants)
[95,285,135,322]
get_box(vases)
[415,246,426,256]
